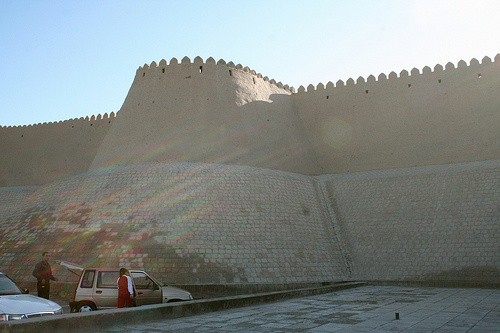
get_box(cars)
[0,271,63,321]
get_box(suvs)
[53,260,195,313]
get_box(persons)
[117,268,136,308]
[32,251,58,300]
